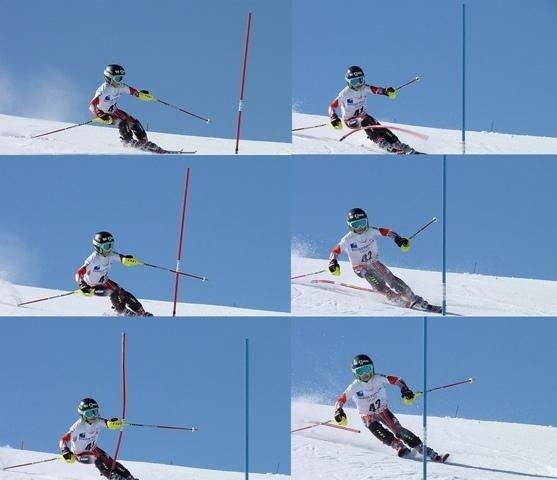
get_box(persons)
[88,63,159,152]
[334,353,438,460]
[75,230,153,317]
[59,398,139,479]
[326,65,415,155]
[328,207,433,310]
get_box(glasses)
[112,75,125,84]
[348,76,364,86]
[353,365,372,376]
[99,242,114,252]
[83,408,98,418]
[349,218,367,229]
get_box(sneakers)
[417,445,438,459]
[121,139,161,150]
[395,442,411,458]
[121,309,153,317]
[407,295,432,311]
[380,140,415,154]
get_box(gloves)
[393,234,411,252]
[384,86,397,98]
[107,417,122,429]
[334,408,349,426]
[140,89,150,94]
[78,281,93,296]
[61,447,77,465]
[328,258,342,276]
[96,111,112,121]
[122,254,137,267]
[400,386,417,406]
[329,114,343,130]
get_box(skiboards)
[120,136,197,155]
[384,301,460,316]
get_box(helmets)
[345,65,364,86]
[104,64,125,84]
[346,207,367,226]
[92,231,115,254]
[351,354,373,370]
[78,398,99,418]
